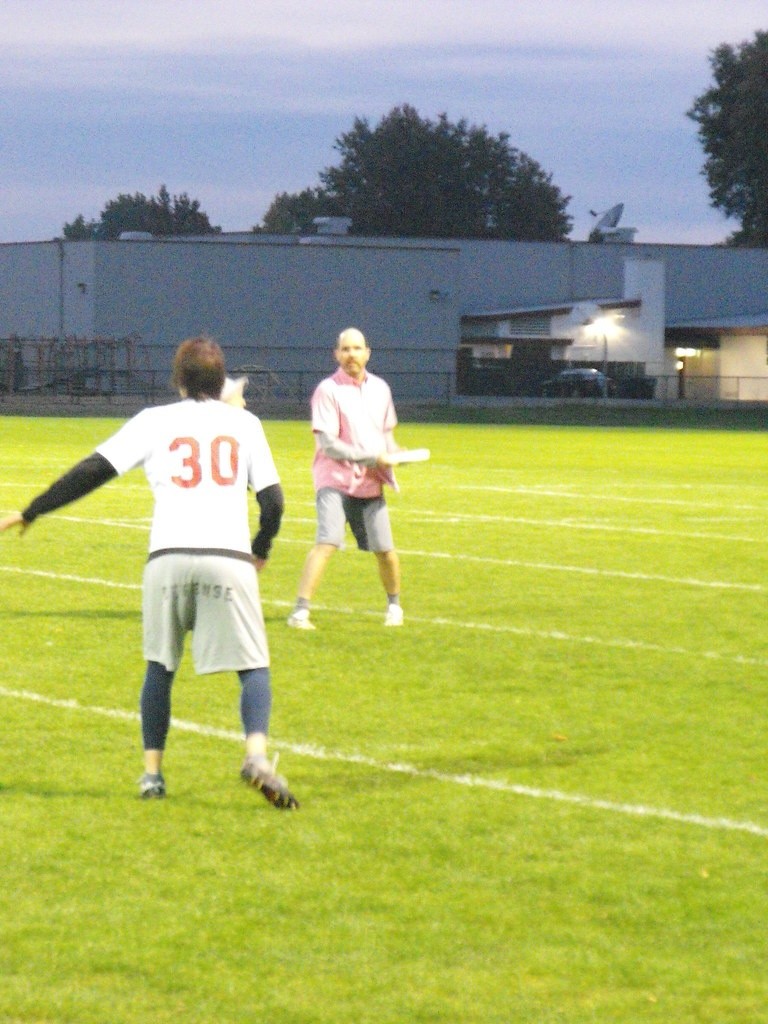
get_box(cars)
[540,369,618,397]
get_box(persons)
[288,326,406,631]
[3,335,300,807]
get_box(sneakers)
[140,772,165,800]
[240,751,299,812]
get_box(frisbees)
[383,447,432,465]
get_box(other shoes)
[385,603,404,626]
[287,609,316,630]
